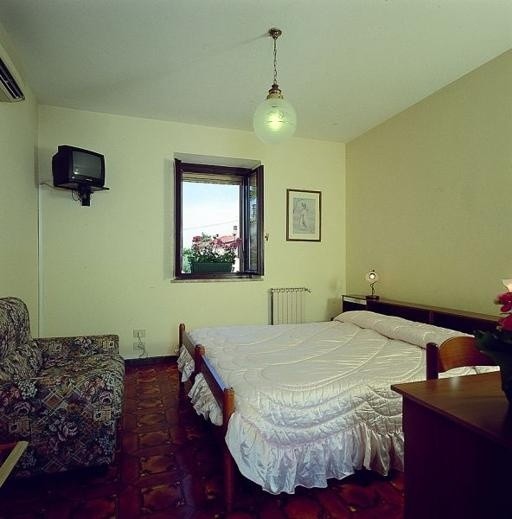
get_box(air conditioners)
[1,19,32,105]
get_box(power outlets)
[133,343,144,351]
[133,330,147,338]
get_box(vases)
[493,332,511,403]
[192,261,233,275]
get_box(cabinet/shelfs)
[390,372,511,517]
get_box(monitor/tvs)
[52,145,105,186]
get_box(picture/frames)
[286,188,322,244]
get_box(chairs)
[424,333,499,380]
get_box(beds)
[173,297,500,517]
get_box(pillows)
[331,307,474,347]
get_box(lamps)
[366,270,379,301]
[252,27,298,149]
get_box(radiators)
[268,288,311,326]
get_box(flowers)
[496,282,512,333]
[188,231,238,262]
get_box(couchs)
[0,296,124,482]
